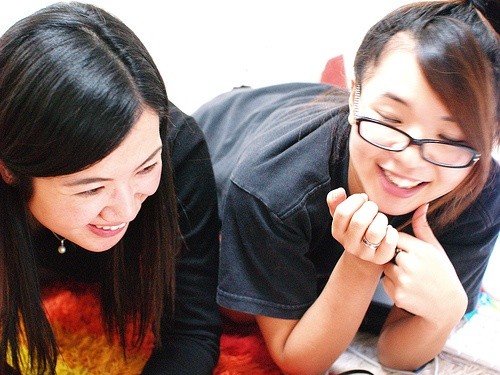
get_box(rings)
[362,236,381,249]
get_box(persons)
[0,1,221,374]
[177,0,500,375]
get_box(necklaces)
[52,231,68,254]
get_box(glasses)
[354,83,480,168]
